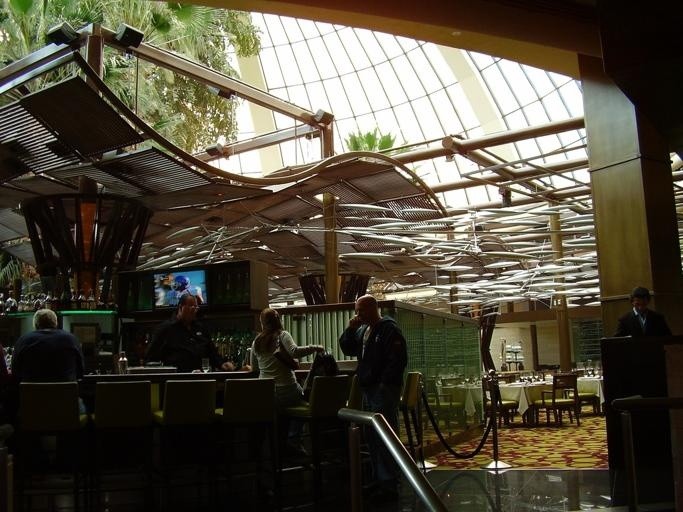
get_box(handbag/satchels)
[303,349,340,403]
[272,335,300,370]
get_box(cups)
[201,357,210,374]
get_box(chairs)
[5,367,422,459]
[424,363,612,435]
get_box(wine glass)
[519,372,543,384]
[586,366,599,378]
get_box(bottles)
[0,288,118,313]
[213,330,254,362]
[118,351,128,373]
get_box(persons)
[9,307,84,466]
[249,307,324,456]
[338,294,407,502]
[144,293,235,409]
[0,343,8,429]
[614,286,673,338]
[173,275,203,305]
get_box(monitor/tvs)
[150,267,210,310]
